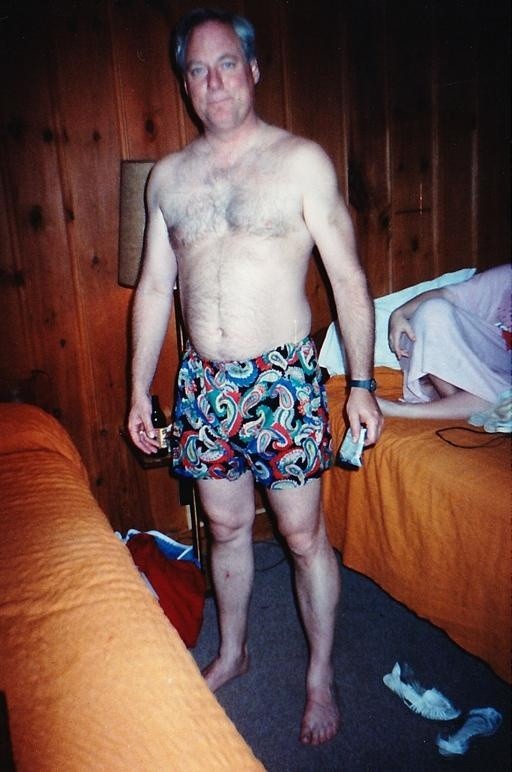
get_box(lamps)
[117,158,219,603]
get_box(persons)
[128,16,382,746]
[378,263,512,420]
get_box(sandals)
[382,659,454,721]
[437,703,503,760]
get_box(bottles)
[151,396,171,458]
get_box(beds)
[313,360,510,681]
[0,401,271,769]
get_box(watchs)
[347,379,377,392]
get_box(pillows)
[316,266,477,377]
